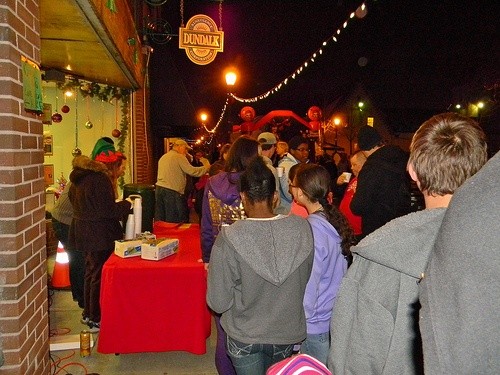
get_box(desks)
[95,220,213,355]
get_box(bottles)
[80,329,90,358]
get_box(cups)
[125,198,142,241]
[277,167,283,177]
[343,172,352,183]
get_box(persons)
[52,137,134,329]
[155,112,500,375]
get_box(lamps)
[64,76,73,97]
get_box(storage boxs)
[114,231,179,261]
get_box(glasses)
[294,148,310,152]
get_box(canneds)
[79,330,90,358]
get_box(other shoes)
[80,312,100,333]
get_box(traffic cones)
[48,240,71,291]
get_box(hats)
[258,132,277,144]
[357,125,381,149]
[169,140,191,150]
